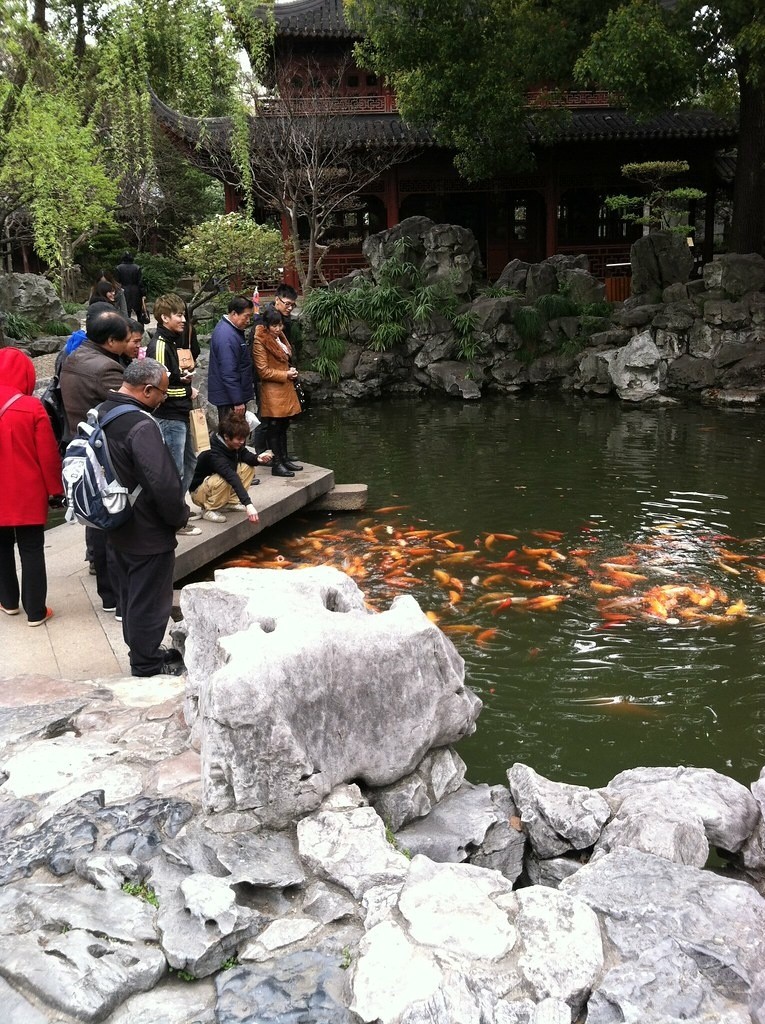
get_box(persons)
[0,346,63,627]
[54,250,303,678]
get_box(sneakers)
[202,511,227,523]
[216,503,245,512]
[176,524,202,535]
[187,512,202,521]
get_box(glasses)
[278,296,297,309]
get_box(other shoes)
[28,607,53,626]
[115,612,123,621]
[0,602,20,614]
[288,455,298,461]
[160,664,187,676]
[103,602,116,611]
[159,648,183,664]
[250,479,260,485]
[89,563,97,575]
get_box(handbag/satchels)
[291,383,312,421]
[141,298,150,324]
[189,397,211,459]
[176,347,195,375]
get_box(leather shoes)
[272,464,295,477]
[283,461,303,471]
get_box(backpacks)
[61,401,165,532]
[40,363,69,445]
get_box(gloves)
[49,495,67,509]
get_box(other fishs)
[211,504,765,646]
